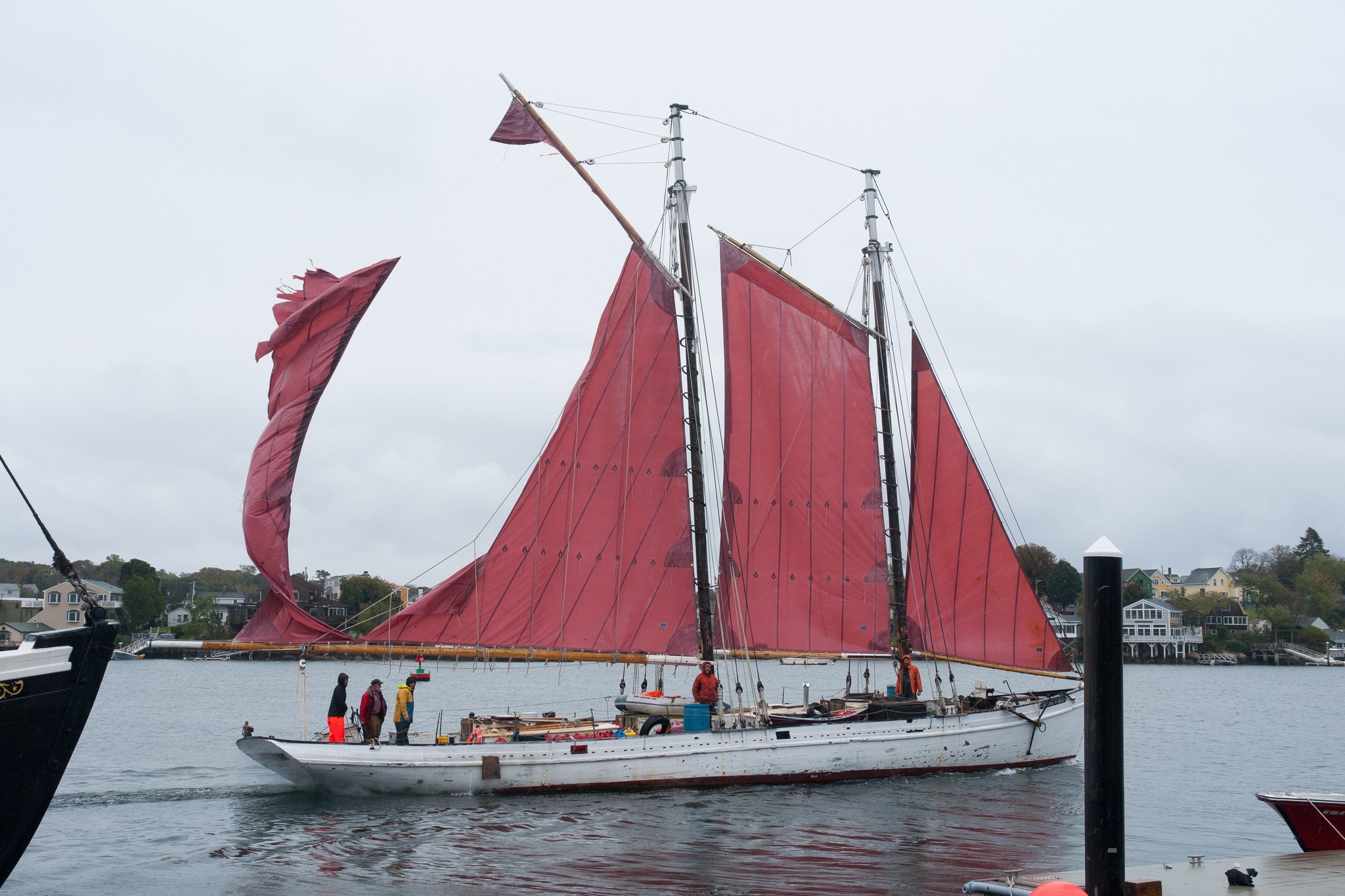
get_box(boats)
[752,705,869,727]
[613,695,699,716]
[1305,642,1345,667]
[1255,790,1345,852]
[0,452,122,884]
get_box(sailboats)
[236,73,1084,799]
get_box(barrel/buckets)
[624,729,636,737]
[604,730,616,737]
[683,703,710,733]
[614,728,627,737]
[545,732,593,751]
[438,736,449,744]
[671,722,684,733]
[886,684,896,696]
[595,731,604,738]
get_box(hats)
[705,663,711,667]
[371,678,384,685]
[410,678,416,683]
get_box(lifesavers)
[639,713,672,737]
[1027,880,1090,896]
[466,727,483,744]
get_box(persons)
[692,661,719,730]
[393,677,416,745]
[327,673,349,742]
[894,654,923,701]
[359,679,388,743]
